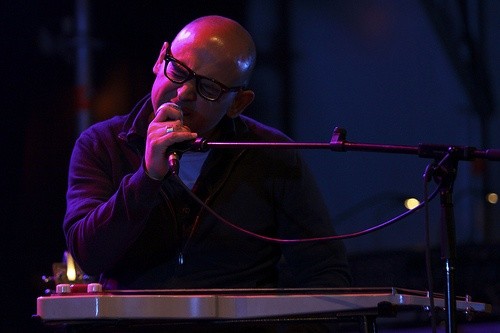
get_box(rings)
[167,127,173,133]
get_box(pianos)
[32,280,493,322]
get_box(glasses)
[164,44,244,101]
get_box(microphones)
[155,102,183,173]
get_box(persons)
[62,16,357,290]
